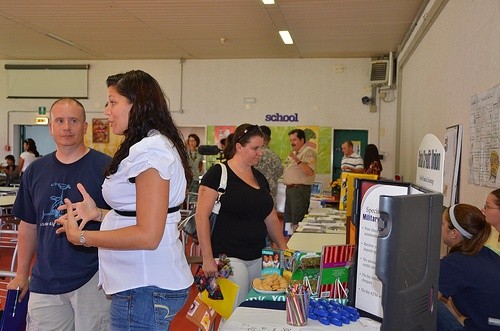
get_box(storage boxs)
[284,249,301,272]
[262,248,282,269]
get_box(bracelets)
[297,161,301,164]
[100,208,103,222]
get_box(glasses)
[238,125,262,140]
[484,204,500,210]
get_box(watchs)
[80,229,91,248]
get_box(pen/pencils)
[284,284,310,327]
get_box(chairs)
[179,192,203,275]
[0,184,204,311]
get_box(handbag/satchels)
[177,163,227,243]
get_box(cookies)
[252,276,289,292]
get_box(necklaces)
[189,147,197,162]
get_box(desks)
[0,195,17,206]
[0,187,19,191]
[219,208,382,331]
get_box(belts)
[286,184,303,188]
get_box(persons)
[340,142,364,173]
[186,134,204,183]
[17,138,41,182]
[481,189,500,257]
[0,155,18,177]
[254,126,283,212]
[342,144,382,176]
[436,204,500,331]
[282,129,316,232]
[53,70,195,331]
[262,254,280,268]
[195,123,288,310]
[7,97,115,331]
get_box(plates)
[250,285,286,295]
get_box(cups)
[284,292,310,326]
